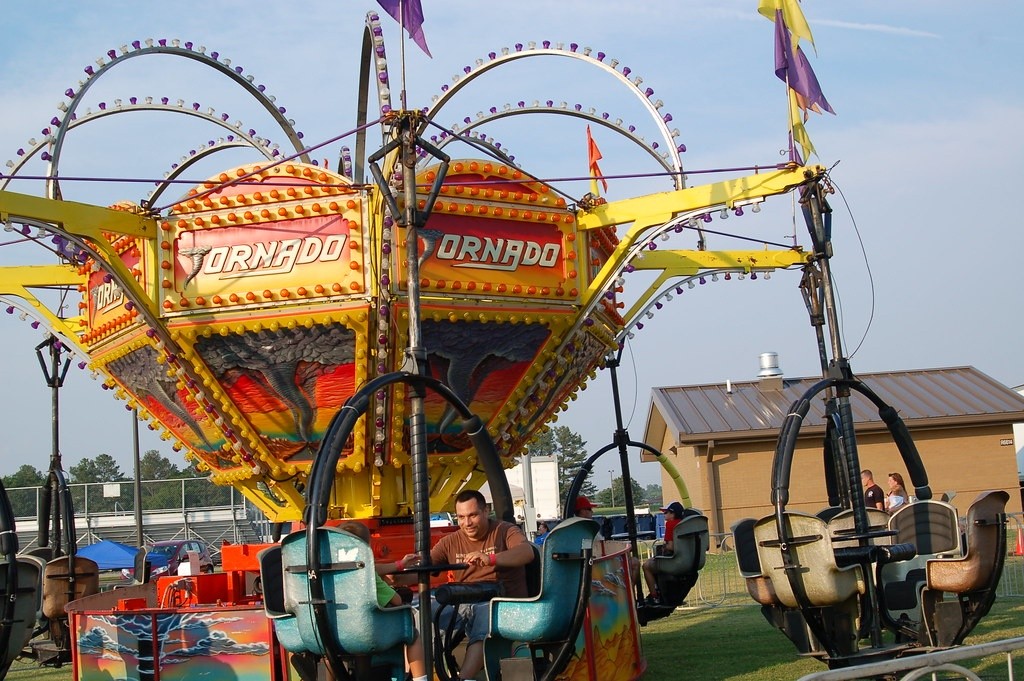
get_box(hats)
[659,501,686,518]
[575,496,597,510]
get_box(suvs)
[120,539,214,584]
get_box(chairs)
[730,489,1010,648]
[256,517,599,681]
[655,509,707,597]
[0,545,99,672]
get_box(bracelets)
[487,554,497,566]
[395,560,404,572]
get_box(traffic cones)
[1007,524,1024,556]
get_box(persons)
[641,502,685,605]
[860,470,909,515]
[335,520,429,681]
[534,523,552,548]
[573,495,640,592]
[430,490,535,681]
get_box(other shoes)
[645,594,662,609]
